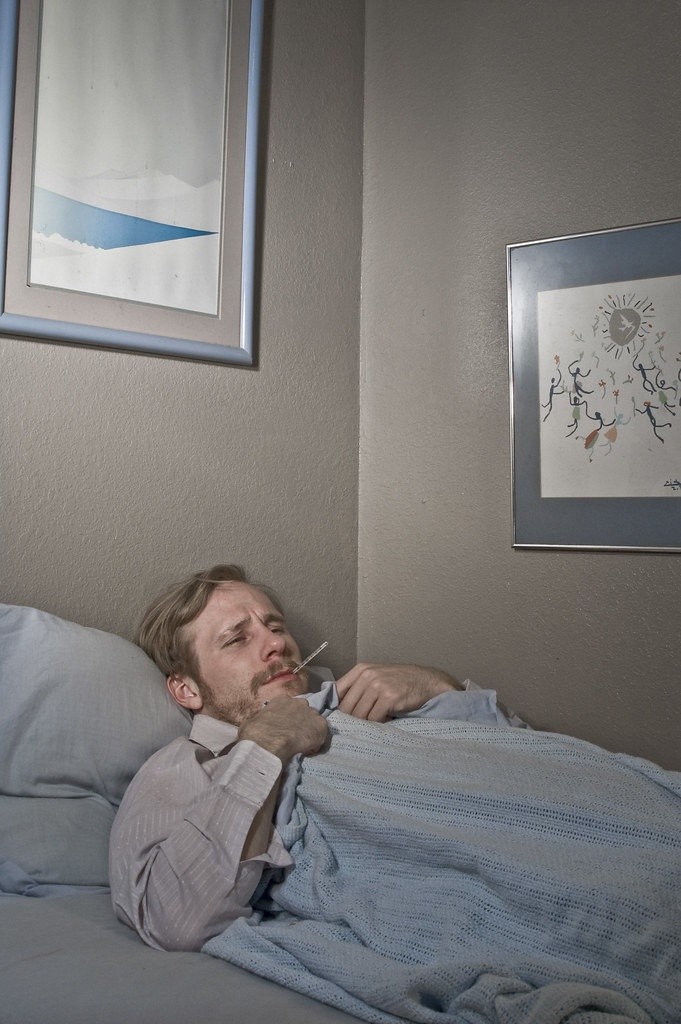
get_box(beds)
[0,601,681,1024]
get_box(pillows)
[0,603,194,896]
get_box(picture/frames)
[0,0,264,367]
[506,218,681,553]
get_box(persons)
[106,563,681,1024]
